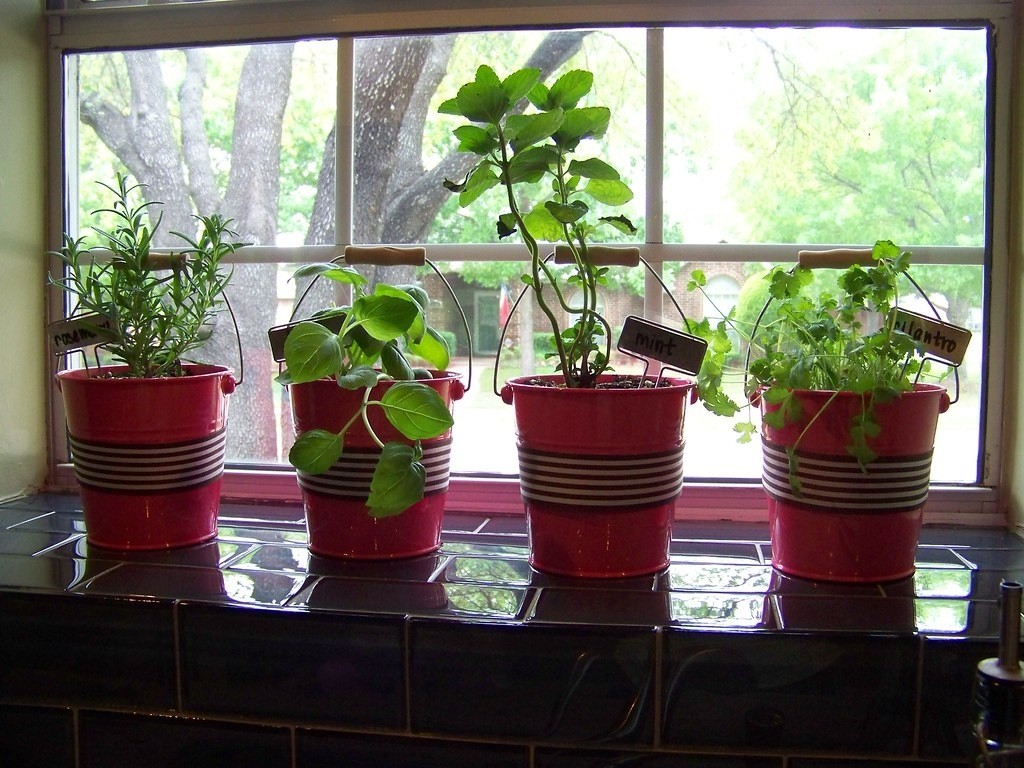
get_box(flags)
[500,285,509,327]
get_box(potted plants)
[702,240,972,583]
[267,246,472,561]
[45,172,244,550]
[436,64,701,579]
